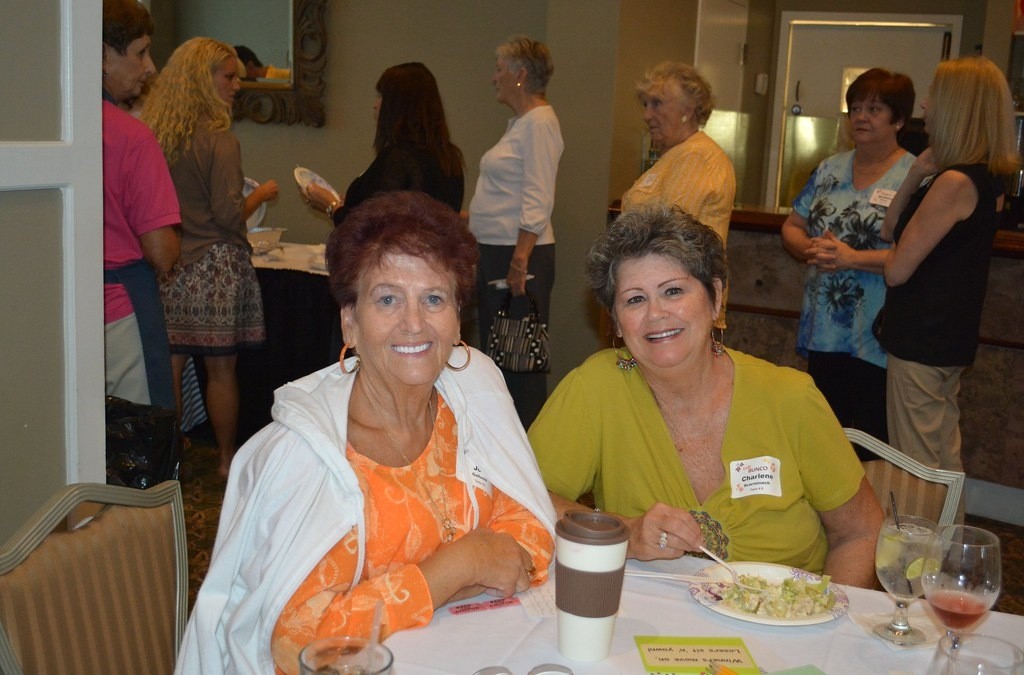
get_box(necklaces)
[357,373,456,542]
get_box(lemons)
[877,534,904,569]
[905,556,942,580]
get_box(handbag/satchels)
[485,296,553,377]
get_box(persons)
[467,36,565,431]
[620,62,736,329]
[782,68,917,485]
[299,62,464,227]
[870,57,1018,543]
[103,0,183,421]
[525,206,884,590]
[233,45,290,81]
[173,190,556,675]
[138,37,280,479]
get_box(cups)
[298,637,396,675]
[932,632,1024,675]
[555,510,629,660]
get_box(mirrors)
[140,0,327,129]
[773,18,950,213]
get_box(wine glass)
[922,526,1001,675]
[873,516,941,647]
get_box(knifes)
[624,569,722,583]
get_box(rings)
[660,533,667,549]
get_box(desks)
[185,241,352,448]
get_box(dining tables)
[362,555,1024,675]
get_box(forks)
[700,547,764,594]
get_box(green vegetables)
[724,575,837,614]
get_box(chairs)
[0,480,189,675]
[841,428,966,599]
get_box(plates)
[293,166,340,205]
[691,559,849,625]
[242,177,266,232]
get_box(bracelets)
[510,262,526,272]
[326,201,343,213]
